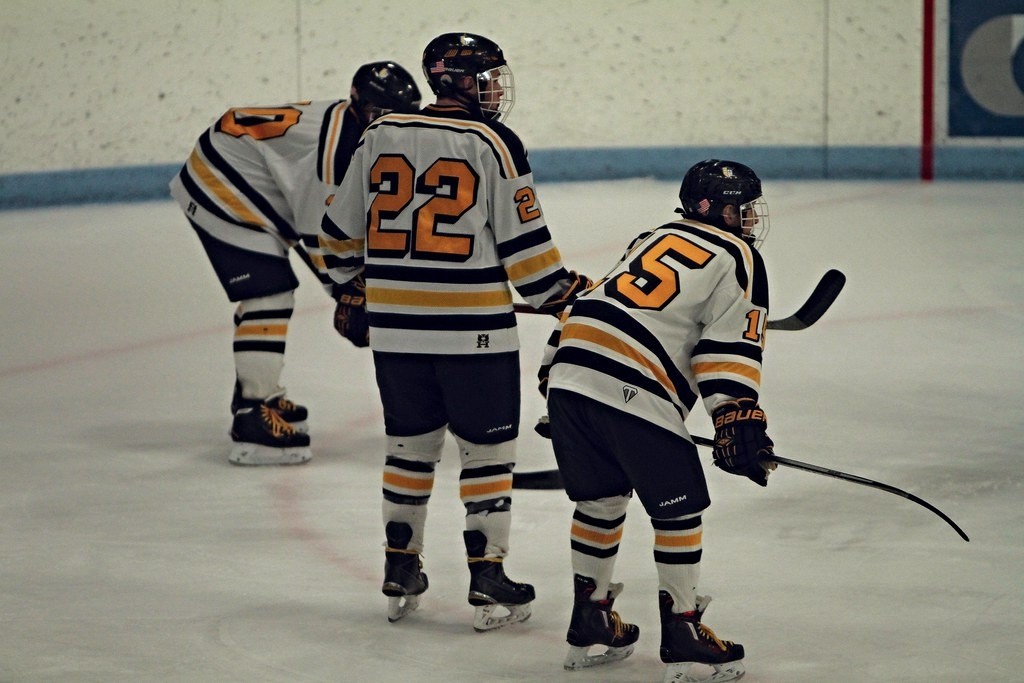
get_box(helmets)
[679,157,771,251]
[349,61,422,128]
[424,31,515,126]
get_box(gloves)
[542,270,595,321]
[332,279,371,348]
[710,398,777,488]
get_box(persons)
[167,61,421,467]
[534,160,779,683]
[318,32,593,634]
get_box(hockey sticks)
[688,433,975,545]
[293,243,567,492]
[511,268,850,333]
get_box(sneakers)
[462,528,534,633]
[659,588,745,683]
[382,520,427,624]
[228,397,314,466]
[229,379,308,433]
[562,573,640,670]
